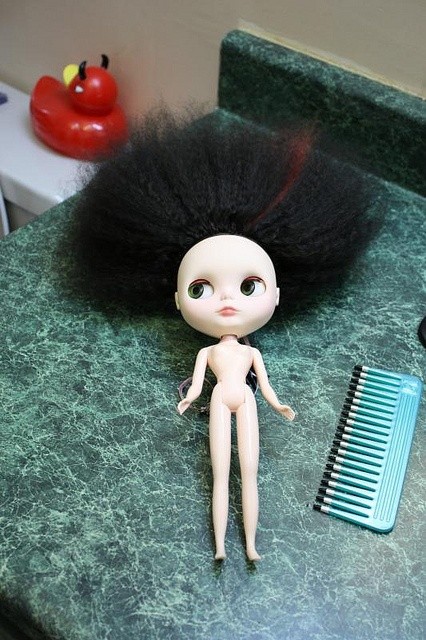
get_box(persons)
[64,100,391,568]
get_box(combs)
[311,362,421,536]
